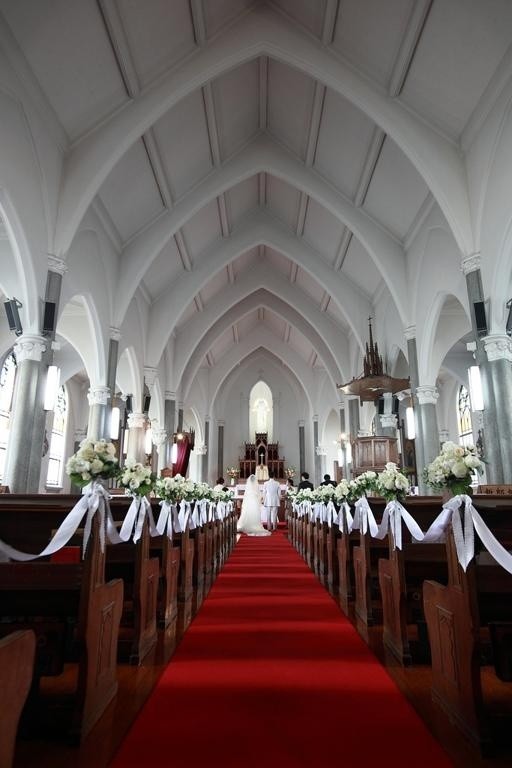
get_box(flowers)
[285,441,489,499]
[65,437,211,507]
[226,464,240,479]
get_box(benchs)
[285,494,511,767]
[0,487,238,768]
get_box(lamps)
[43,364,61,412]
[467,365,484,411]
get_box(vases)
[230,478,235,486]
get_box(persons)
[298,472,314,492]
[281,479,297,529]
[320,475,337,488]
[235,474,272,537]
[263,472,282,532]
[221,487,228,491]
[214,478,225,492]
